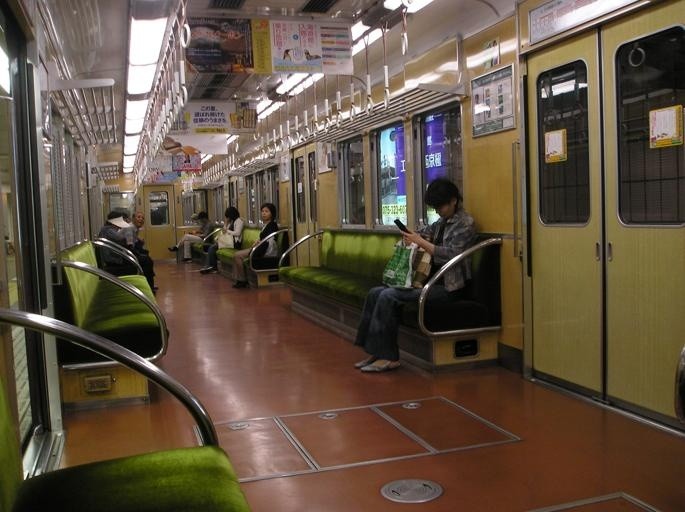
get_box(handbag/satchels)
[412,247,432,289]
[218,230,234,249]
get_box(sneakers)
[200,265,218,274]
[168,246,179,252]
[181,258,192,263]
[232,280,250,288]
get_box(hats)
[108,216,130,228]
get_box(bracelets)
[414,233,421,243]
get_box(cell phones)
[393,219,411,235]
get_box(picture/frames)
[470,62,517,138]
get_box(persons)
[97,209,159,294]
[200,206,245,274]
[232,203,282,290]
[353,177,482,374]
[167,211,214,262]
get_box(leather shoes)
[354,359,401,372]
